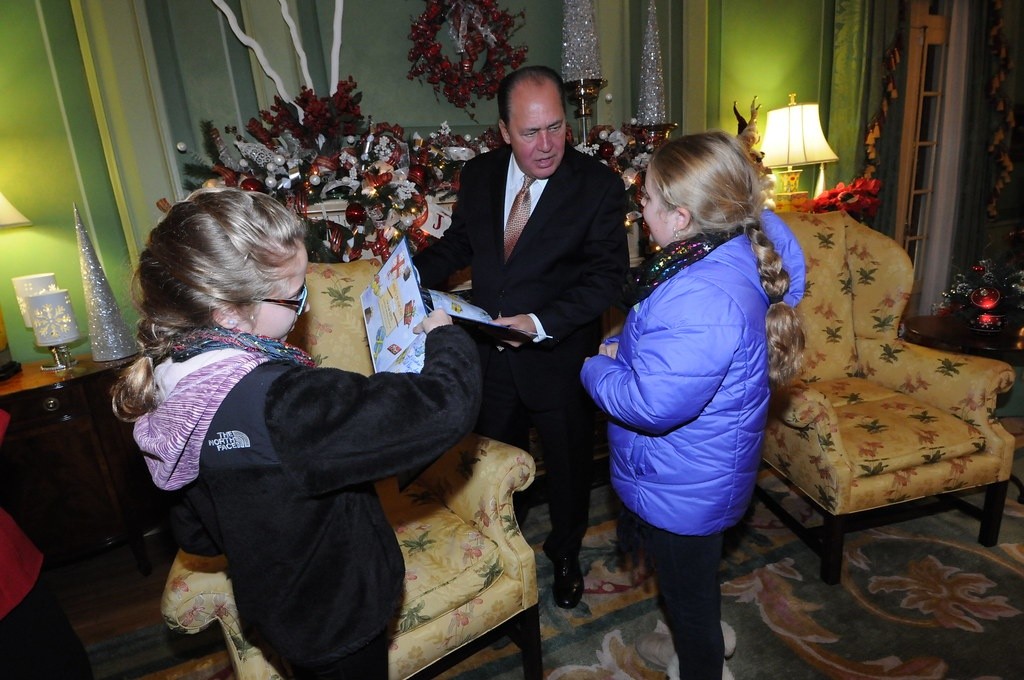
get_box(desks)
[900,313,1023,375]
[1,349,164,566]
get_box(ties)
[504,174,537,263]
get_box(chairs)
[711,211,1016,588]
[160,256,544,680]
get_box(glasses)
[252,277,309,316]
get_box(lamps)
[0,191,36,382]
[10,271,81,371]
[760,93,840,210]
[25,290,86,376]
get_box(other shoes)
[636,619,737,671]
[664,653,736,680]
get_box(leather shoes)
[552,553,585,609]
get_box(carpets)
[67,435,1024,679]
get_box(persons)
[580,128,805,679]
[0,407,94,680]
[410,65,632,610]
[107,186,482,679]
